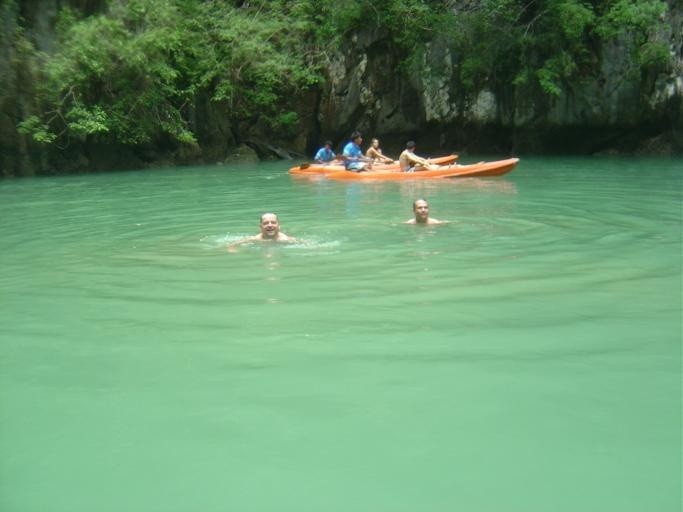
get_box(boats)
[292,158,520,183]
[420,153,460,164]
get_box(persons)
[400,197,448,225]
[226,213,295,253]
[399,140,456,172]
[365,137,395,164]
[313,140,338,164]
[374,148,386,161]
[342,130,381,171]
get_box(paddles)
[335,154,394,163]
[300,161,330,169]
